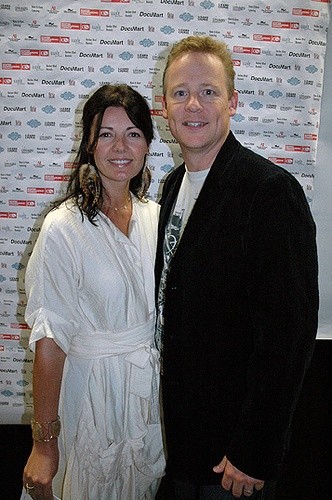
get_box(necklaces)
[100,192,131,211]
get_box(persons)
[154,35,319,500]
[20,83,166,500]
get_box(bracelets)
[31,415,62,442]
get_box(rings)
[26,483,34,490]
